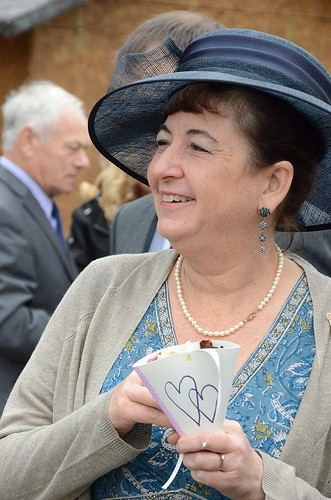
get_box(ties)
[51,202,67,255]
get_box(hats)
[87,29,331,232]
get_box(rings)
[216,453,224,471]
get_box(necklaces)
[174,241,284,337]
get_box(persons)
[66,158,152,272]
[109,10,331,279]
[0,81,92,420]
[0,28,331,500]
